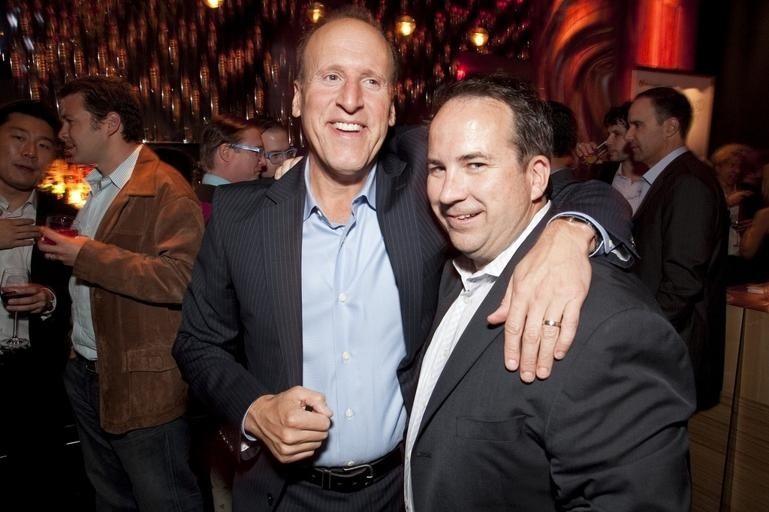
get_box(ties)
[406,274,494,511]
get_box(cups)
[40,215,82,262]
[580,142,613,168]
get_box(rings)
[544,320,562,328]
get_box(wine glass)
[0,267,31,350]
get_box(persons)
[0,100,79,512]
[171,8,640,512]
[192,114,268,227]
[539,101,578,177]
[37,78,205,512]
[402,76,698,512]
[247,117,299,178]
[623,86,731,485]
[711,142,759,287]
[575,100,649,218]
[740,164,769,284]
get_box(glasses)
[263,146,298,165]
[228,143,265,159]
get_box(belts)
[70,348,97,374]
[290,439,405,495]
